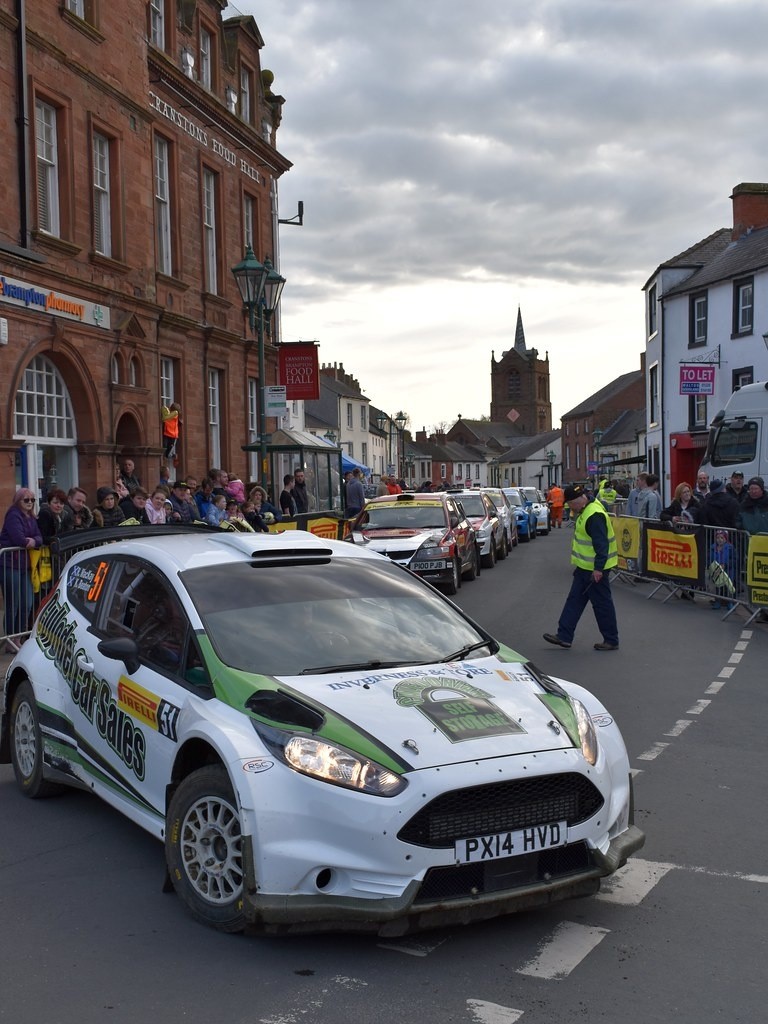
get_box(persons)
[162,403,181,469]
[708,530,736,611]
[387,475,402,494]
[599,477,608,492]
[660,482,704,600]
[0,488,43,654]
[635,474,658,583]
[577,484,589,500]
[564,485,577,521]
[635,473,664,523]
[285,601,340,672]
[376,475,392,496]
[438,483,451,492]
[596,482,623,511]
[347,469,367,517]
[289,469,308,513]
[726,471,749,594]
[280,474,299,514]
[692,472,710,503]
[342,471,354,517]
[702,479,741,606]
[437,481,447,491]
[545,483,565,528]
[422,481,432,493]
[543,483,620,651]
[39,458,283,601]
[617,478,627,498]
[626,472,650,518]
[741,477,768,622]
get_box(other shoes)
[595,641,619,650]
[543,633,571,647]
[6,635,29,653]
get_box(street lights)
[592,426,604,490]
[406,449,415,490]
[545,450,557,489]
[233,240,287,495]
[395,411,407,482]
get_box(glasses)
[21,498,36,502]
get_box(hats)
[710,479,725,494]
[173,481,190,489]
[382,475,391,480]
[732,471,744,477]
[715,529,729,540]
[748,477,764,490]
[563,485,585,505]
[226,498,237,505]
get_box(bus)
[695,381,768,491]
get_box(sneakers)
[727,603,735,610]
[713,601,722,609]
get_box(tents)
[316,436,370,479]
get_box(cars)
[0,533,646,938]
[520,487,551,535]
[346,492,479,595]
[444,489,507,568]
[502,488,537,542]
[470,487,519,551]
